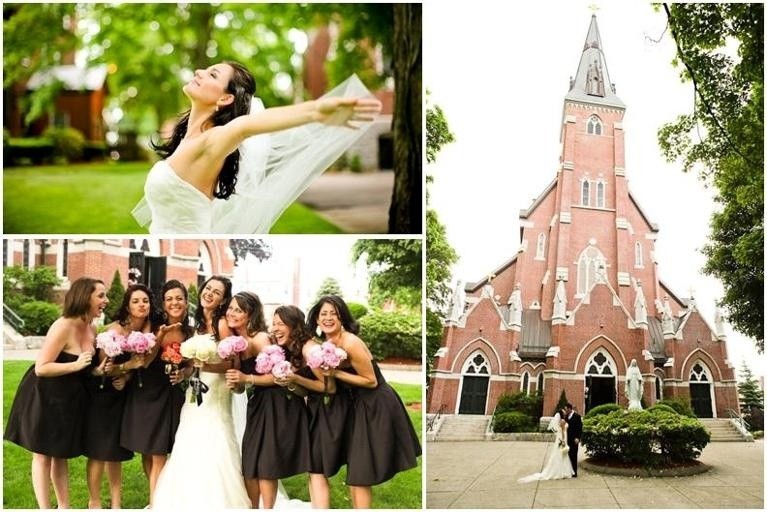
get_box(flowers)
[218,335,249,393]
[160,342,188,396]
[307,342,348,406]
[180,332,217,404]
[124,330,158,388]
[95,329,126,389]
[256,344,299,401]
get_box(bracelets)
[119,362,129,376]
[287,384,298,391]
[247,372,256,388]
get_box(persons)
[269,302,350,509]
[564,403,583,478]
[142,58,385,234]
[307,292,422,509]
[552,407,569,479]
[153,273,241,510]
[118,277,196,509]
[82,281,184,508]
[2,276,115,509]
[624,356,645,411]
[224,289,311,510]
